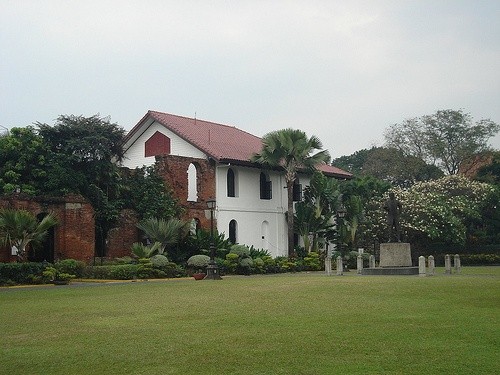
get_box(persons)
[383,192,402,243]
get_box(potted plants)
[52,273,76,285]
[187,255,210,281]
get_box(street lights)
[204,196,224,280]
